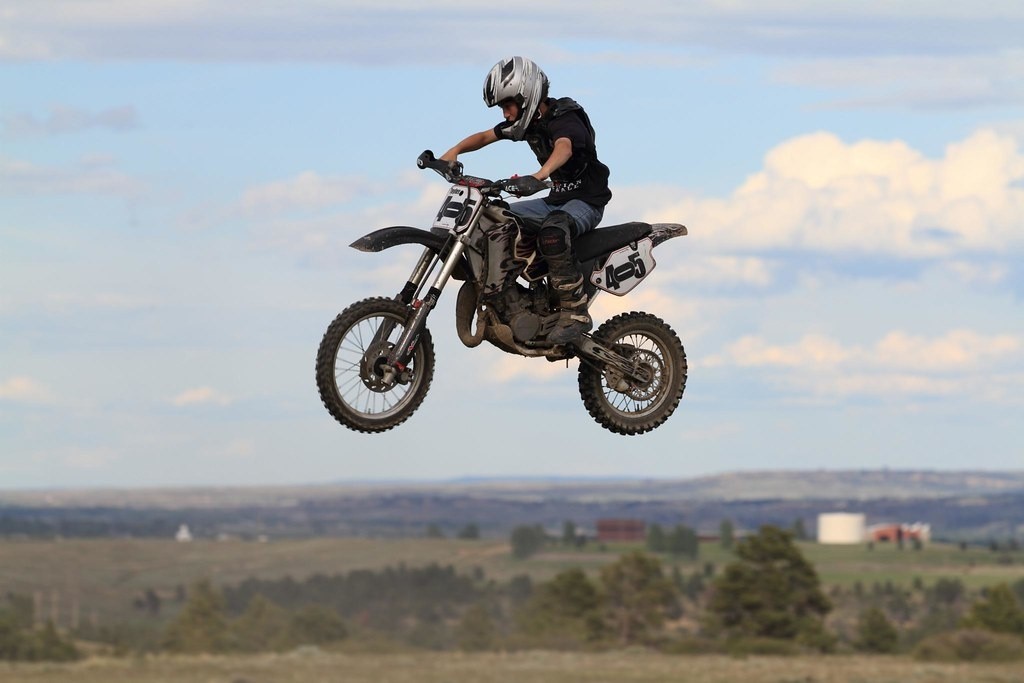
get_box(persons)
[439,56,612,343]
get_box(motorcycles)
[315,150,689,436]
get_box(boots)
[545,272,594,344]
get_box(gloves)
[501,175,544,199]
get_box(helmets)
[482,55,547,141]
